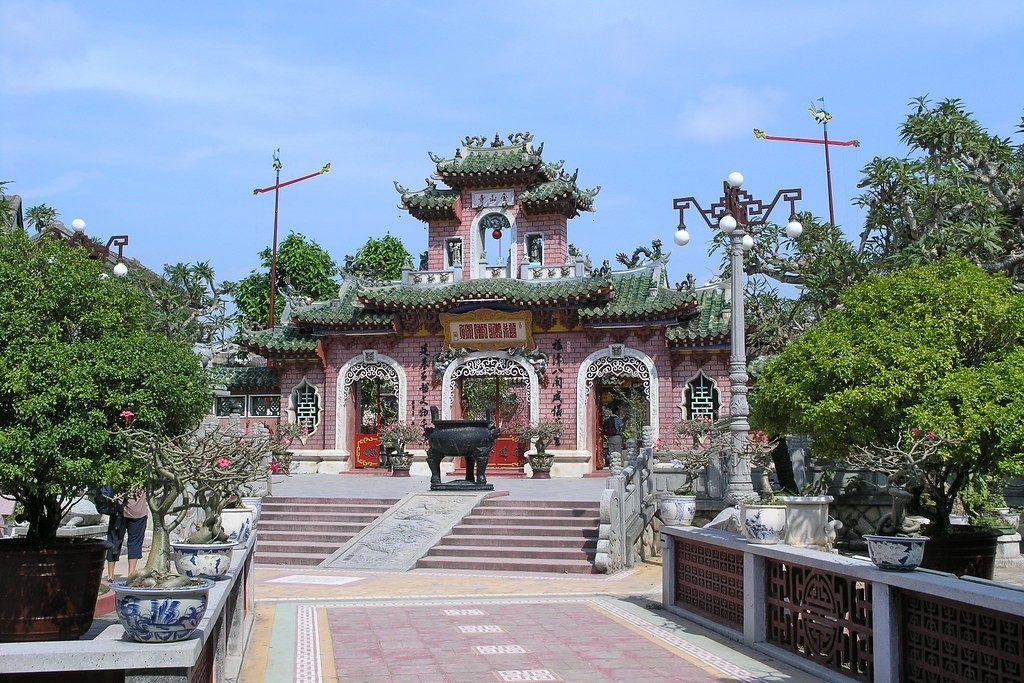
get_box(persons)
[107,489,148,583]
[531,238,541,262]
[450,243,458,263]
[608,407,623,453]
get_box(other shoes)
[108,579,115,587]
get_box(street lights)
[673,172,803,505]
[222,300,229,341]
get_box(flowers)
[104,411,311,588]
[852,423,963,536]
[653,413,726,496]
[376,416,428,454]
[712,429,781,503]
[505,417,561,455]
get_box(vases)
[862,535,930,573]
[0,536,113,642]
[170,540,238,581]
[659,495,696,526]
[272,452,293,474]
[920,523,1005,579]
[528,454,554,479]
[993,508,1019,533]
[221,507,253,550]
[388,453,414,477]
[109,577,216,643]
[242,496,262,529]
[740,503,787,545]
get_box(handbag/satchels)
[92,482,122,516]
